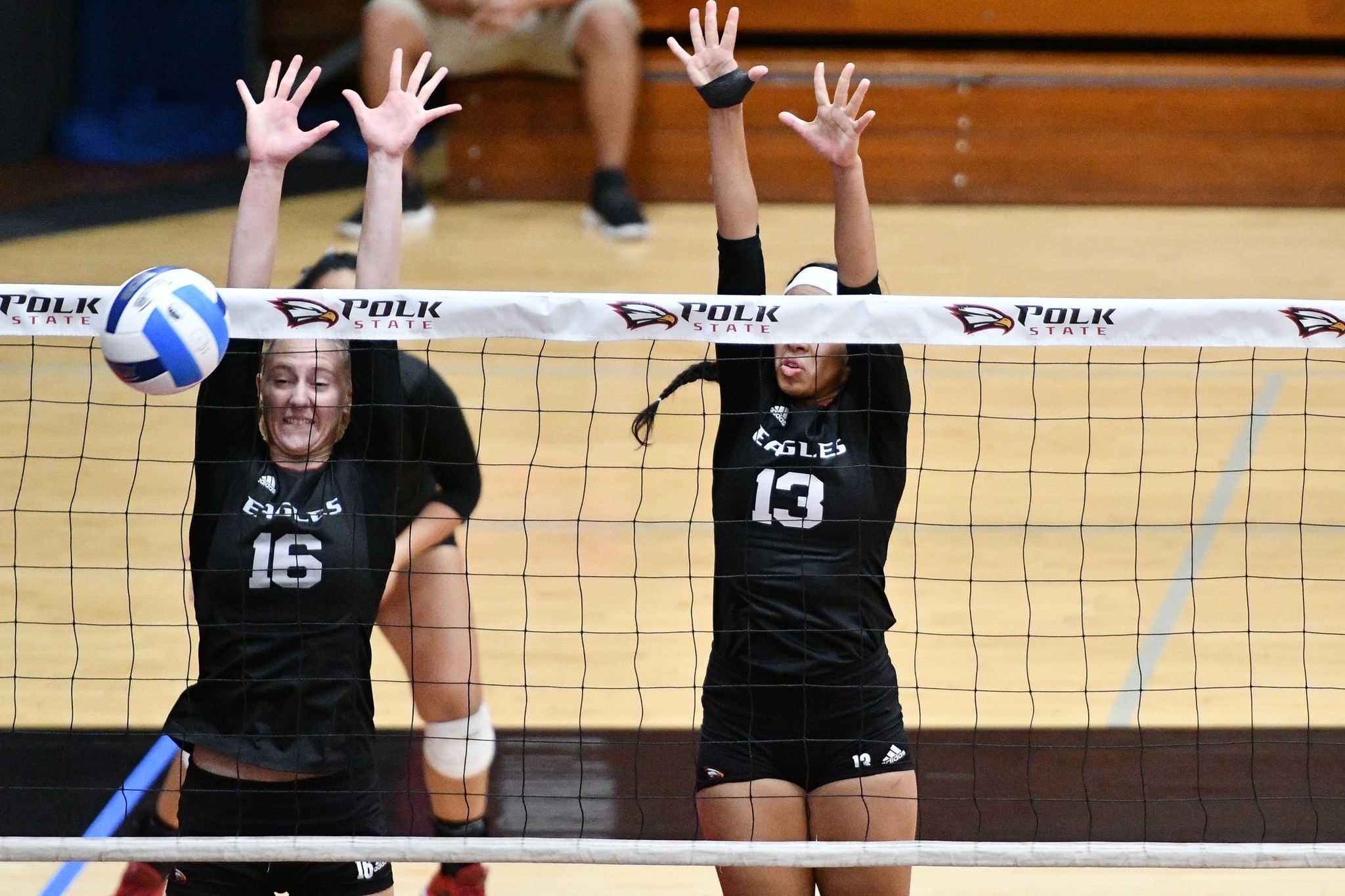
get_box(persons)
[161,48,463,896]
[336,0,653,239]
[665,1,919,896]
[117,253,498,896]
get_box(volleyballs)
[99,266,230,396]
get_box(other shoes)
[339,169,433,239]
[116,861,167,896]
[426,863,486,896]
[583,165,651,238]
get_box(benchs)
[442,0,1345,209]
